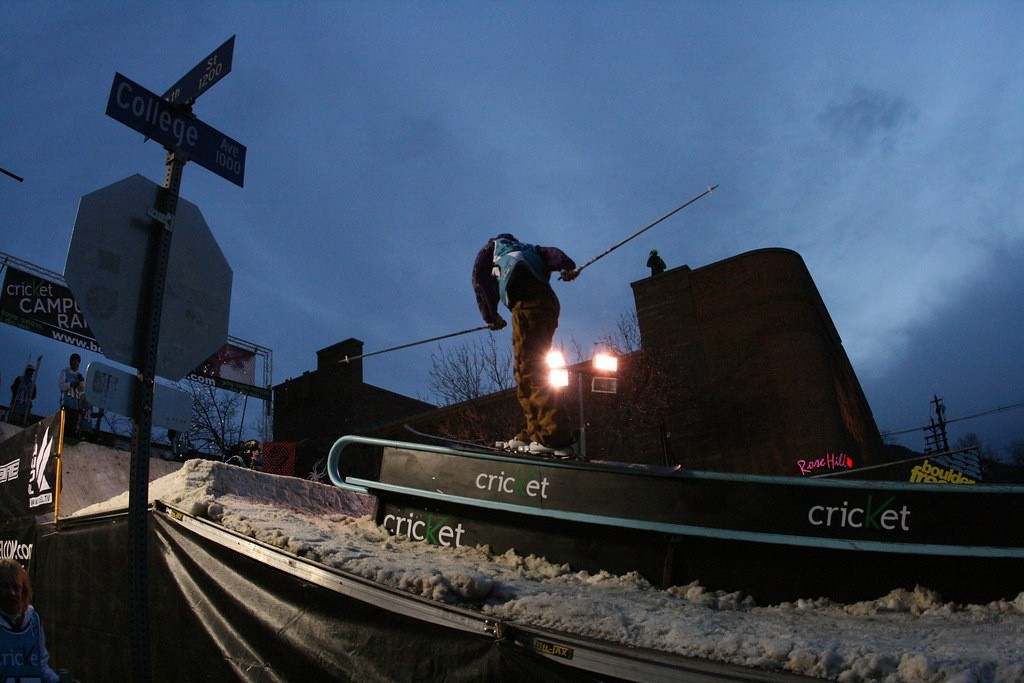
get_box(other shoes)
[518,441,575,461]
[503,437,531,454]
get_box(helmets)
[244,440,270,451]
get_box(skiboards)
[402,421,684,475]
[7,352,45,429]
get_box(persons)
[0,559,58,683]
[8,365,37,428]
[168,430,195,462]
[59,353,103,445]
[472,233,578,459]
[228,440,261,470]
